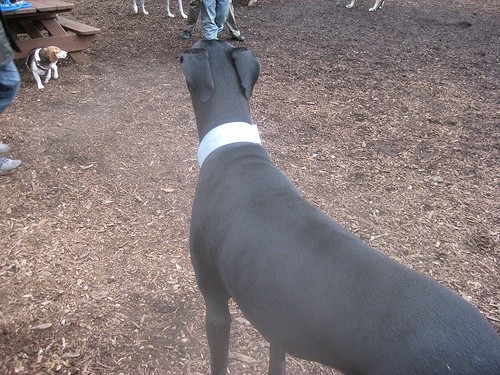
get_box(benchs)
[55,15,100,35]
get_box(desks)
[0,0,91,66]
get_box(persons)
[200,0,232,40]
[181,0,246,41]
[0,18,22,173]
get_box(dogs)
[346,0,385,12]
[25,45,69,90]
[131,0,188,19]
[179,39,500,375]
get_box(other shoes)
[0,142,10,153]
[0,158,22,171]
[181,30,190,39]
[233,35,246,41]
